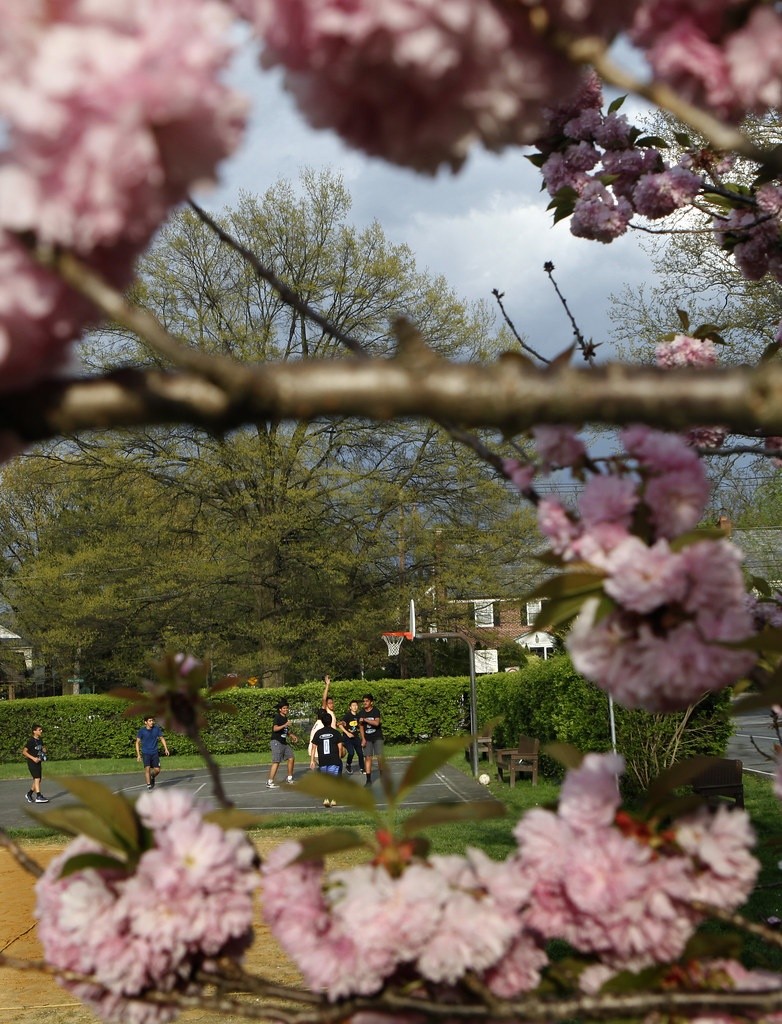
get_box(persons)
[308,709,328,773]
[136,715,169,793]
[338,700,366,775]
[309,713,343,807]
[23,725,49,804]
[265,702,298,789]
[321,674,346,759]
[357,693,392,787]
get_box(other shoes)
[364,780,372,788]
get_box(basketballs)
[338,746,346,758]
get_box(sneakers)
[35,794,48,802]
[322,799,330,806]
[345,764,353,774]
[24,793,33,803]
[265,780,280,788]
[146,785,153,793]
[285,778,296,785]
[359,769,366,774]
[330,799,337,806]
[150,775,155,789]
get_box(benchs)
[495,735,539,788]
[679,755,744,815]
[465,725,493,763]
